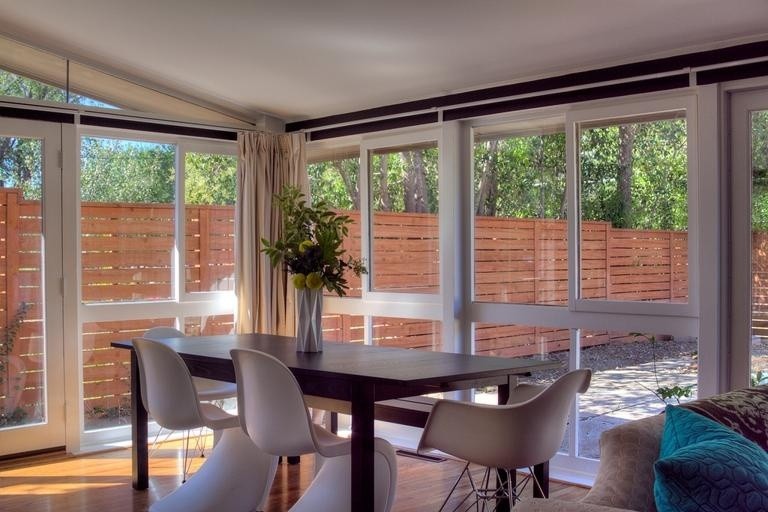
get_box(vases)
[295,287,323,353]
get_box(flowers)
[260,182,369,298]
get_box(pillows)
[653,404,768,512]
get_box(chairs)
[230,348,397,512]
[417,367,592,512]
[143,327,237,484]
[132,337,280,512]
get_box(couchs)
[509,383,768,512]
